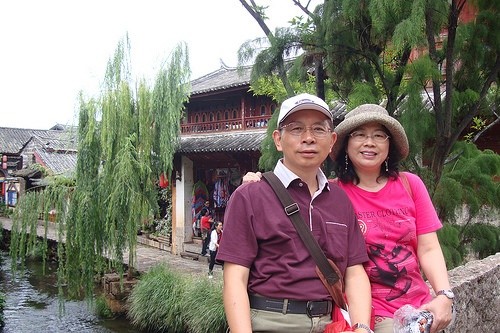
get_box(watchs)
[436,290,454,299]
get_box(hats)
[277,93,333,129]
[329,104,409,164]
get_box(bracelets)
[352,323,371,333]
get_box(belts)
[247,293,332,318]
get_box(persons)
[215,93,372,333]
[200,208,214,257]
[242,104,453,333]
[198,200,213,227]
[208,221,223,279]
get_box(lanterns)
[159,175,168,189]
[3,156,7,169]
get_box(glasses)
[348,131,392,143]
[278,122,332,137]
